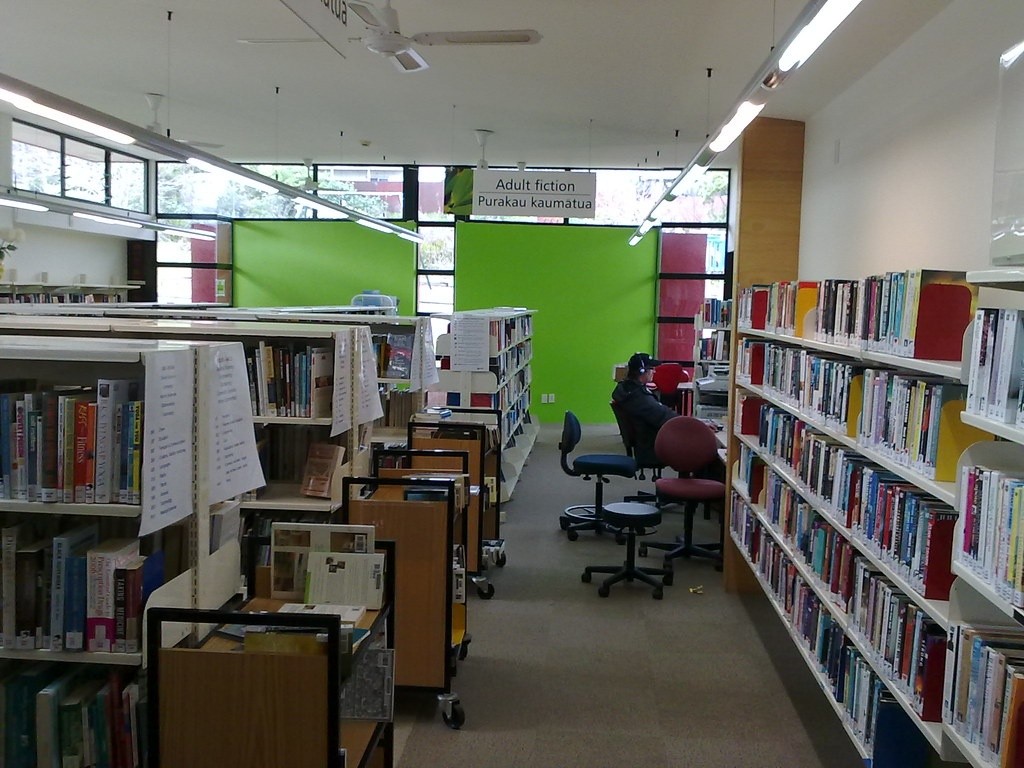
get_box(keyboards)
[703,419,724,431]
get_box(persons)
[612,354,725,485]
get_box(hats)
[628,353,662,371]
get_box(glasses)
[642,366,655,370]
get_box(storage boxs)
[613,364,628,381]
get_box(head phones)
[636,352,647,375]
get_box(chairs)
[653,362,690,411]
[558,411,636,545]
[608,400,686,510]
[638,416,725,571]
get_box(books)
[694,268,1024,768]
[0,292,531,768]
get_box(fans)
[244,0,537,74]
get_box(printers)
[695,364,730,406]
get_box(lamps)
[0,74,423,243]
[627,1,856,246]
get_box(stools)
[581,503,672,599]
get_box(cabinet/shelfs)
[0,271,540,768]
[730,270,1024,767]
